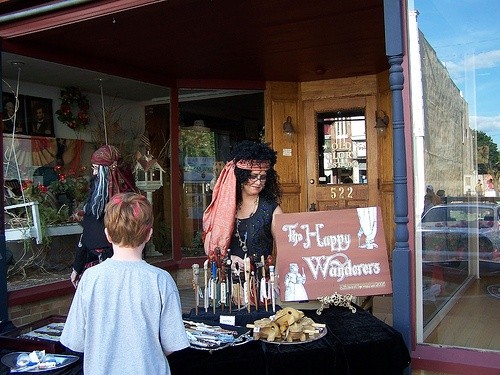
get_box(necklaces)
[236,195,260,253]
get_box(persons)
[475,178,484,197]
[423,184,441,209]
[486,178,496,196]
[436,190,447,203]
[71,144,143,290]
[202,141,284,284]
[59,191,191,375]
[343,177,353,184]
[3,101,24,134]
[31,105,51,135]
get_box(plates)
[262,327,327,344]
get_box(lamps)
[374,109,389,134]
[283,116,294,137]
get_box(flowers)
[20,165,89,207]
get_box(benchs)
[4,179,83,244]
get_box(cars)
[422,203,500,277]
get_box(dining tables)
[166,305,412,375]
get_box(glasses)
[248,174,267,180]
[91,166,98,170]
